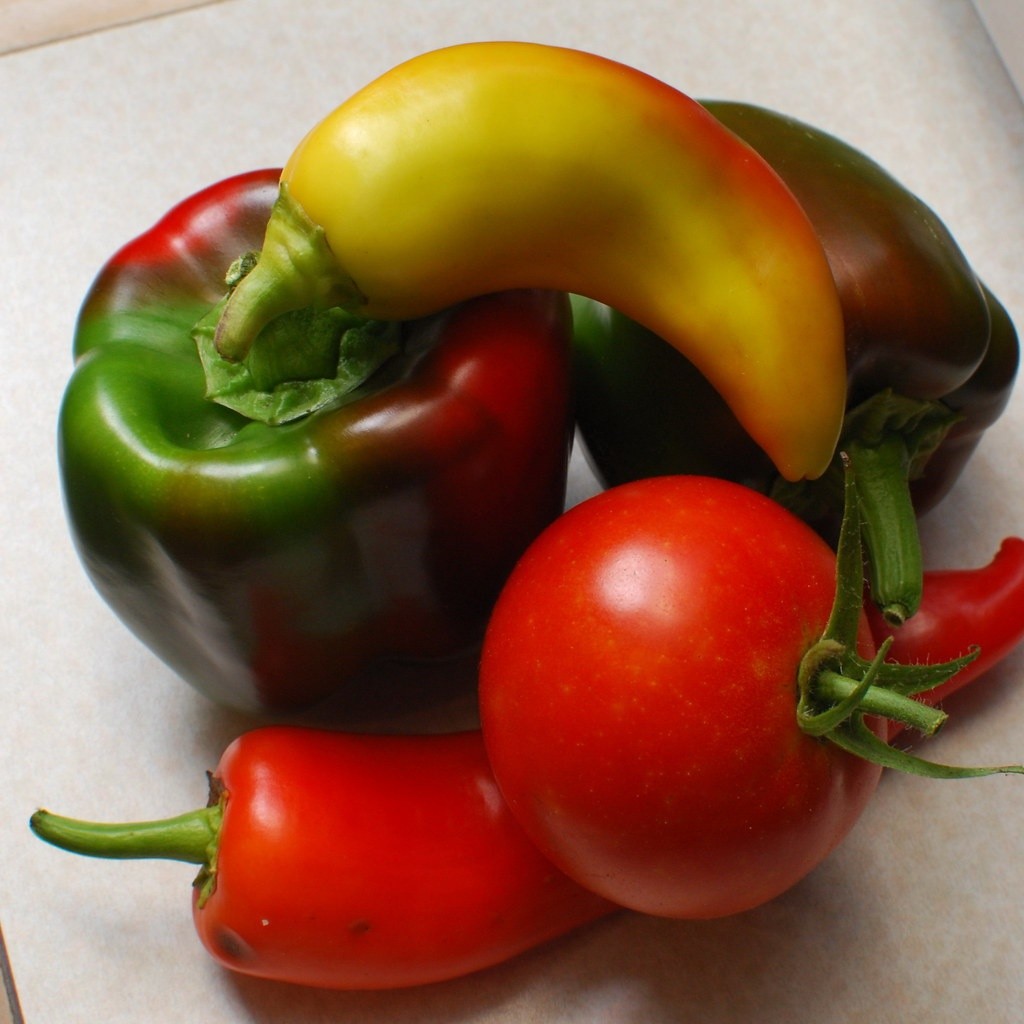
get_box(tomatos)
[477,449,1023,918]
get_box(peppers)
[27,39,1024,990]
